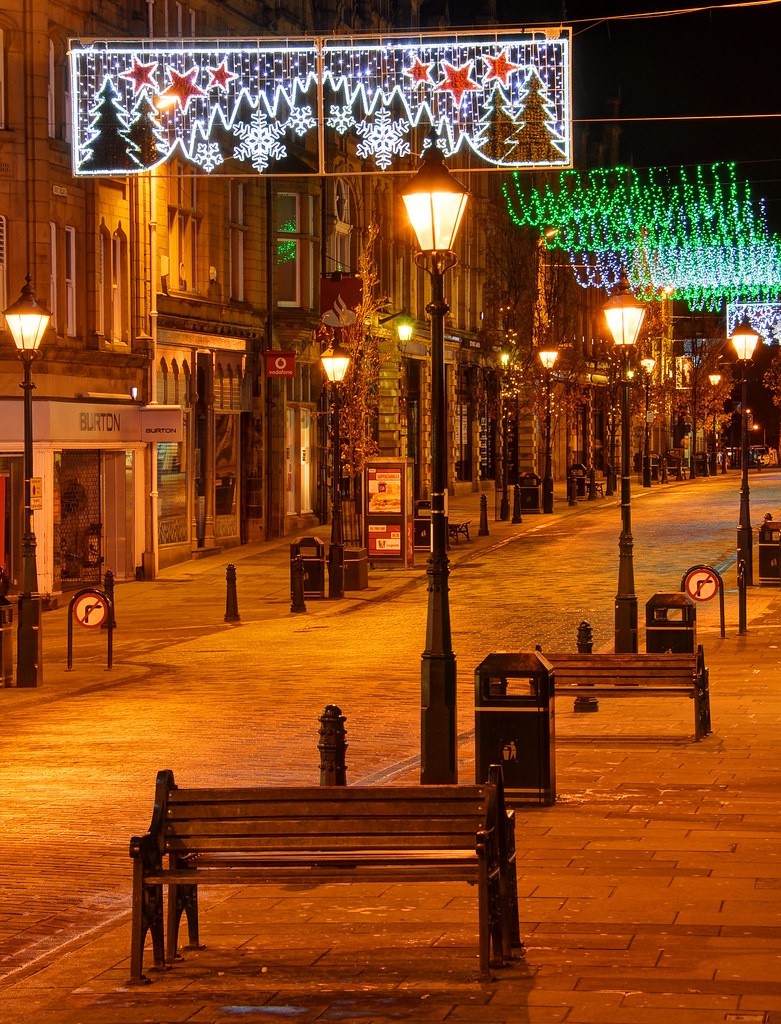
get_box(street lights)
[538,341,558,514]
[727,308,763,591]
[0,274,59,691]
[639,349,656,488]
[706,365,721,476]
[318,328,353,600]
[395,129,473,784]
[598,264,651,686]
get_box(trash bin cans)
[644,593,698,654]
[290,536,325,600]
[758,520,780,587]
[413,500,432,552]
[475,651,556,807]
[518,471,543,514]
[570,463,587,497]
[695,451,705,477]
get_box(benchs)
[527,643,714,743]
[128,763,525,980]
[449,518,472,543]
[585,481,605,497]
